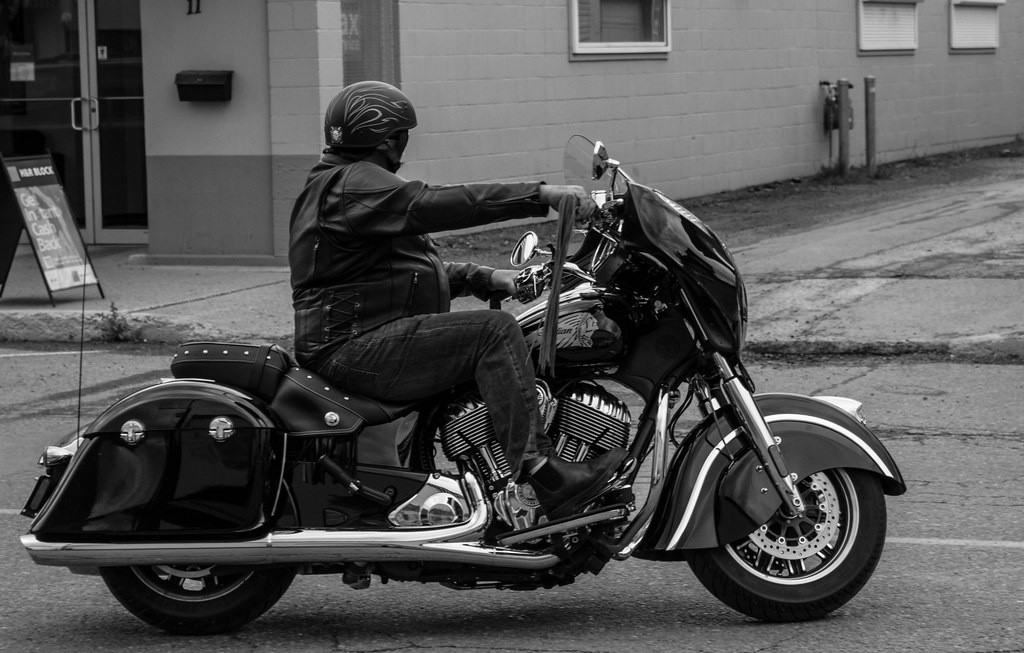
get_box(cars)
[22,26,143,118]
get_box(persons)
[284,78,627,522]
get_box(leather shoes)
[527,446,631,521]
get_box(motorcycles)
[20,132,906,635]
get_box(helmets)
[324,80,418,149]
